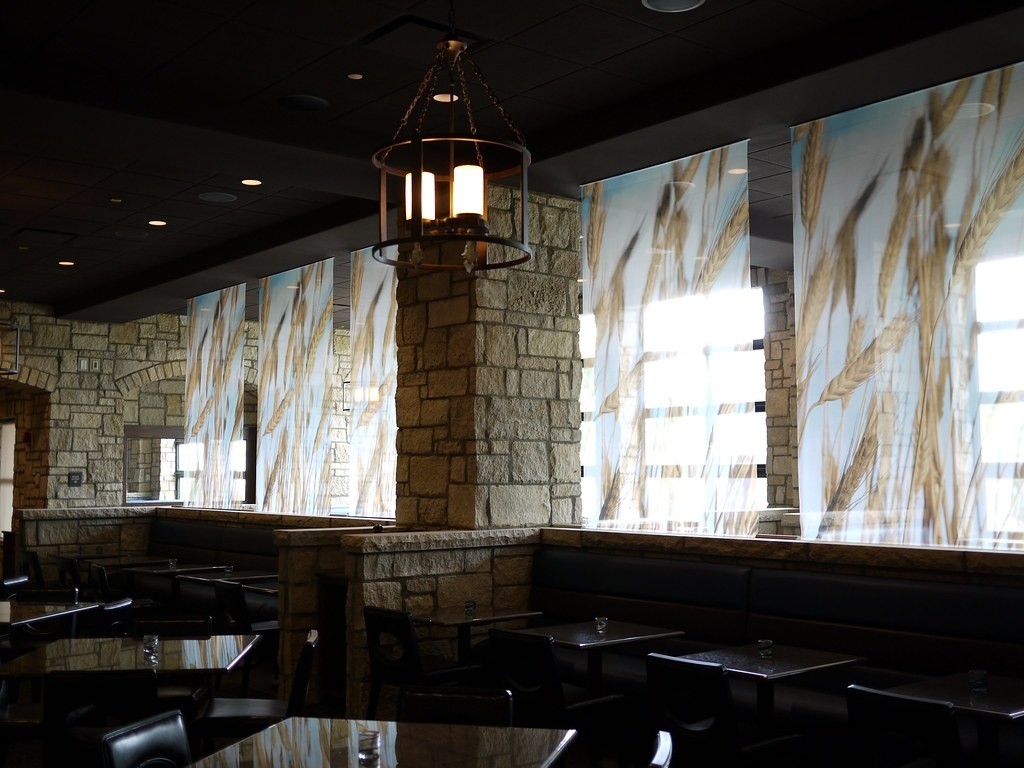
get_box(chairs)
[0,555,840,768]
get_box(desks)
[0,634,264,698]
[241,582,279,591]
[413,608,542,665]
[676,642,862,742]
[132,563,231,574]
[181,714,579,768]
[47,552,128,583]
[848,671,1024,768]
[191,570,279,582]
[517,620,686,699]
[83,558,178,568]
[0,601,89,630]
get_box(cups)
[595,617,608,633]
[969,670,987,691]
[143,635,158,653]
[758,640,773,659]
[358,731,380,760]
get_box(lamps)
[372,39,531,274]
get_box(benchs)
[147,520,280,573]
[524,547,1024,768]
